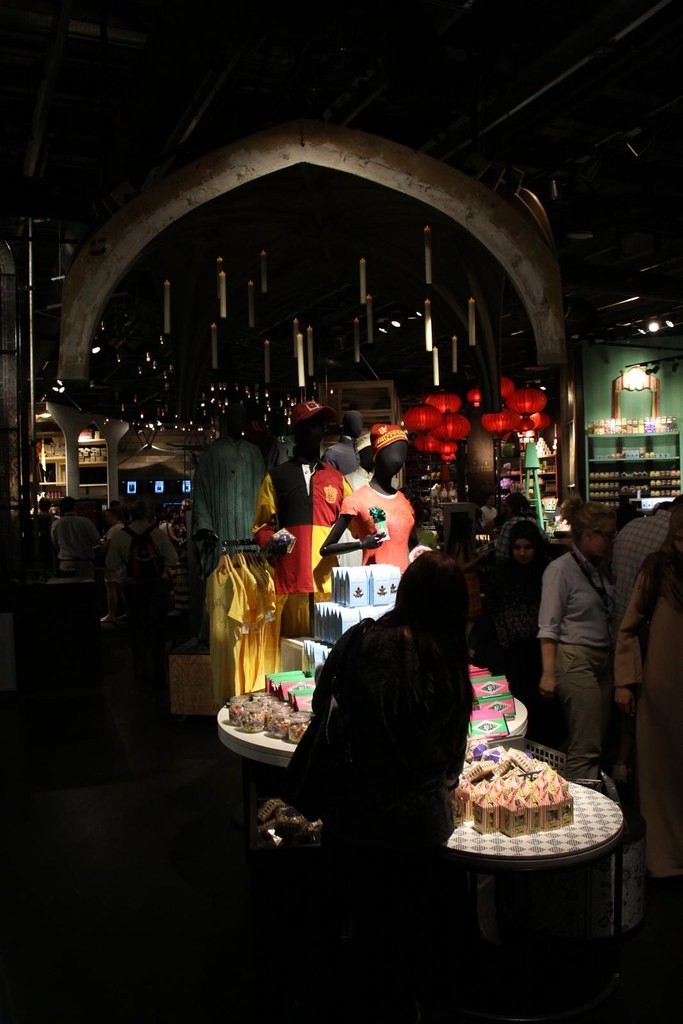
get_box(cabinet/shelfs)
[38,439,107,487]
[403,437,556,543]
[589,463,681,511]
[588,431,683,463]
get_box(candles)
[164,224,475,386]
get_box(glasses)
[592,529,617,542]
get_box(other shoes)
[116,615,128,620]
[167,611,180,616]
[100,616,116,624]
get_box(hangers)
[216,537,266,572]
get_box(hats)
[290,401,337,426]
[370,423,409,464]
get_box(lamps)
[646,362,681,376]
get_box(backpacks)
[118,524,165,581]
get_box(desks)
[217,689,527,875]
[443,778,624,1024]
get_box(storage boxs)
[302,564,402,676]
[447,662,574,838]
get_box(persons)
[320,424,430,575]
[255,402,346,594]
[418,490,683,1023]
[320,551,475,1024]
[39,495,189,680]
[195,402,268,581]
[322,410,364,476]
[341,430,375,491]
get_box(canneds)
[226,691,312,743]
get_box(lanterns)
[405,378,552,460]
